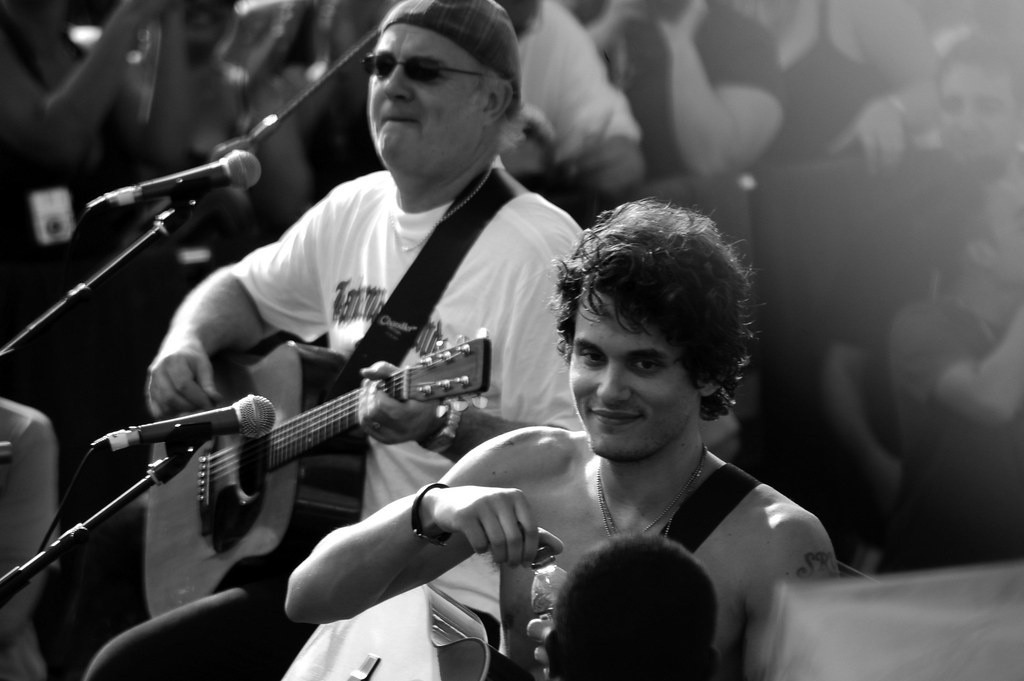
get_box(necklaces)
[596,442,707,540]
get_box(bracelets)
[412,482,453,546]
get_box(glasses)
[363,54,487,83]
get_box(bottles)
[531,544,568,621]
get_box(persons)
[0,0,1024,424]
[285,203,834,681]
[888,155,1024,573]
[543,532,719,681]
[147,0,582,522]
[824,35,1024,572]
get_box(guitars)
[144,327,492,619]
[280,582,536,681]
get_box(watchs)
[418,397,462,453]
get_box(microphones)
[90,394,277,456]
[87,149,262,212]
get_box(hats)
[381,0,520,111]
